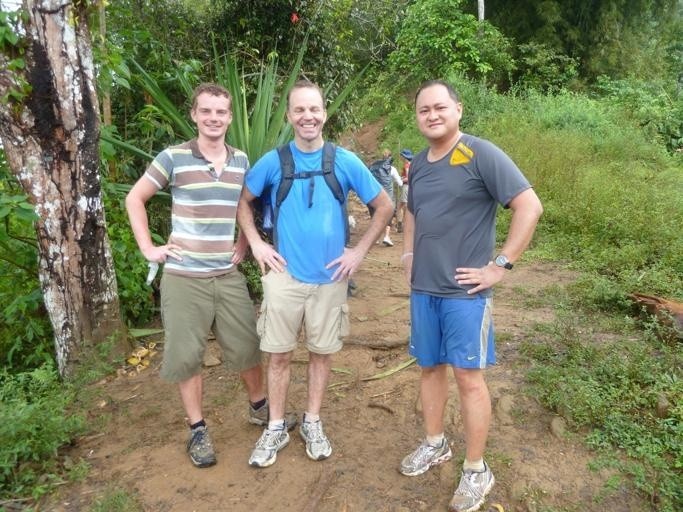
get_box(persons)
[396,82,543,512]
[125,81,298,469]
[236,80,396,468]
[396,150,414,233]
[367,148,403,246]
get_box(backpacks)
[369,156,393,198]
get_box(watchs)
[495,254,514,270]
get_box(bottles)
[260,191,275,231]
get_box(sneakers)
[248,424,290,467]
[398,437,452,477]
[299,420,332,461]
[248,398,297,431]
[382,237,393,246]
[448,459,495,512]
[187,424,217,468]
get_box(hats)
[399,150,413,160]
